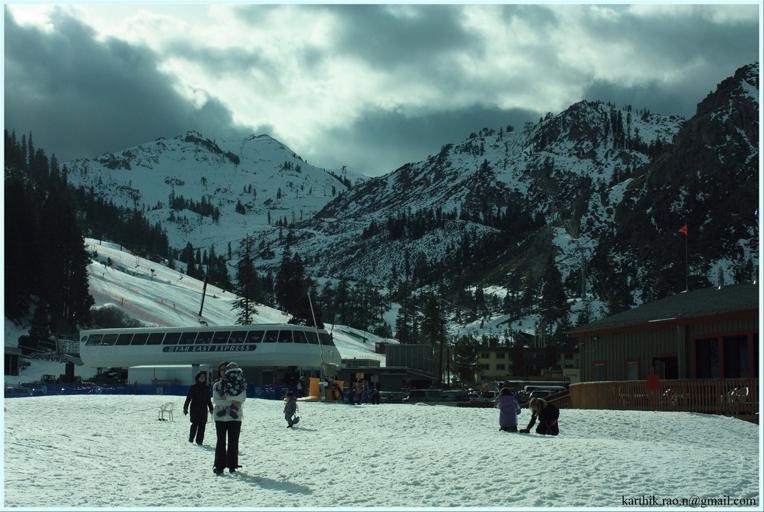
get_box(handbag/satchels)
[292,416,299,424]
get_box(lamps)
[592,334,599,342]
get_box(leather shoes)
[213,466,224,474]
[229,466,236,473]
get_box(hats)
[226,362,240,370]
[287,391,294,396]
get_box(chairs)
[157,402,174,421]
[720,386,749,412]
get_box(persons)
[367,384,380,404]
[215,362,243,468]
[282,392,296,428]
[519,396,560,437]
[496,385,521,432]
[356,377,363,405]
[212,362,247,475]
[184,370,213,444]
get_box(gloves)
[183,409,188,415]
[210,409,213,414]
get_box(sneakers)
[287,424,293,428]
[188,437,203,445]
[229,410,239,419]
[217,410,226,417]
